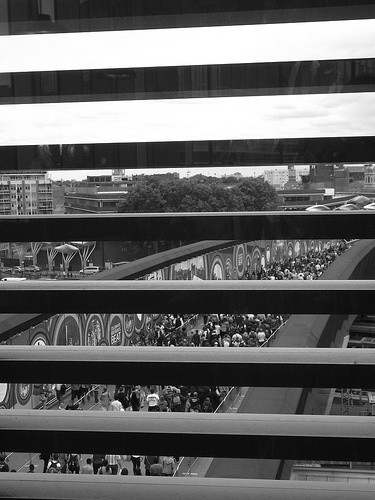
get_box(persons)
[1,236,375,481]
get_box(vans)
[1,277,28,281]
[80,266,102,275]
